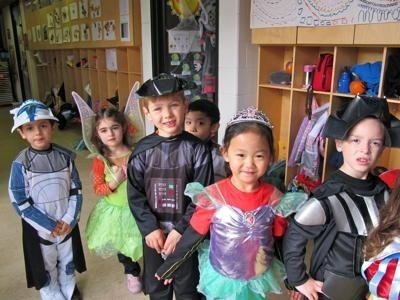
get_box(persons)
[127,73,215,300]
[184,99,230,184]
[85,108,144,294]
[360,174,400,299]
[282,97,392,299]
[155,107,288,300]
[8,100,86,300]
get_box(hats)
[320,92,399,150]
[131,71,198,99]
[8,97,61,134]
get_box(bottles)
[337,72,351,94]
[301,64,313,90]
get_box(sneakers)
[124,272,144,294]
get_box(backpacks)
[311,52,335,93]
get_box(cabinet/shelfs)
[249,0,399,227]
[27,0,142,133]
